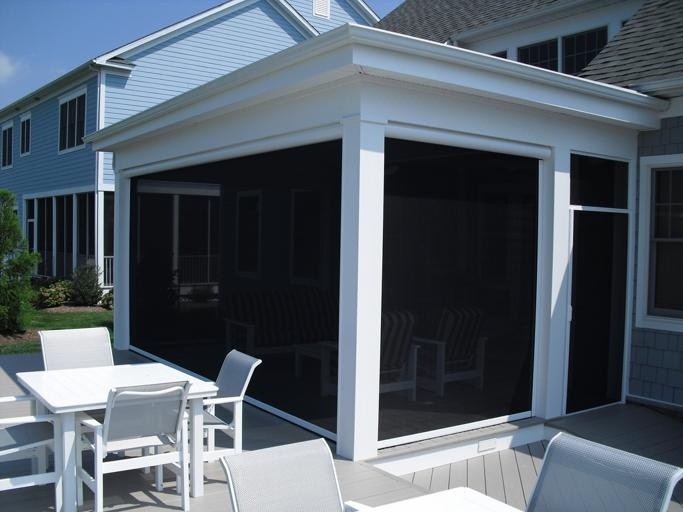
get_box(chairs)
[0,395,62,512]
[226,283,486,401]
[219,437,373,512]
[183,349,262,462]
[37,326,114,370]
[525,432,683,512]
[74,379,193,512]
